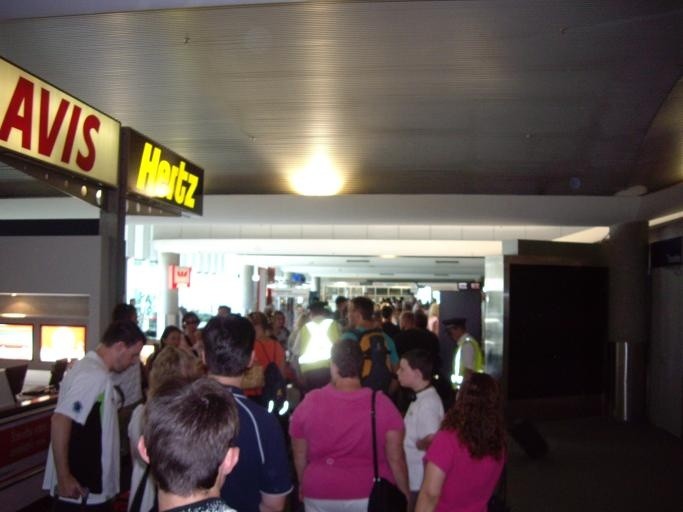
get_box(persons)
[43,291,509,512]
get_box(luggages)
[503,415,550,461]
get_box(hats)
[442,317,467,337]
[306,301,327,310]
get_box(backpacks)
[256,339,291,418]
[346,326,394,391]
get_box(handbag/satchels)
[366,476,408,512]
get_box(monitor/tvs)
[6,364,29,396]
[49,358,68,388]
